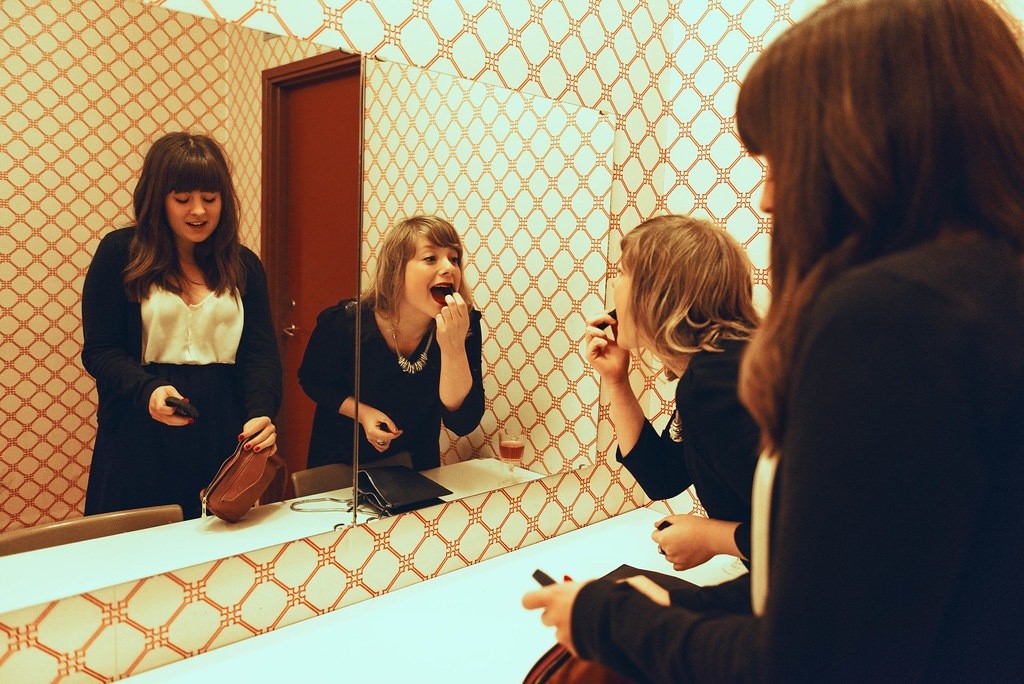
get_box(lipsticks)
[595,308,618,330]
[450,286,456,295]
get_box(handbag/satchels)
[201,437,290,522]
[356,465,454,516]
[522,643,636,684]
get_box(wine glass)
[498,430,528,487]
[718,557,745,576]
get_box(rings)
[658,545,664,554]
[378,439,386,445]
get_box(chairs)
[0,503,184,557]
[289,452,415,497]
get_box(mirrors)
[0,0,361,616]
[354,54,617,527]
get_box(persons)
[297,215,485,473]
[80,128,283,521]
[586,214,766,578]
[522,0,1024,684]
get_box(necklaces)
[389,320,433,374]
[669,411,681,442]
